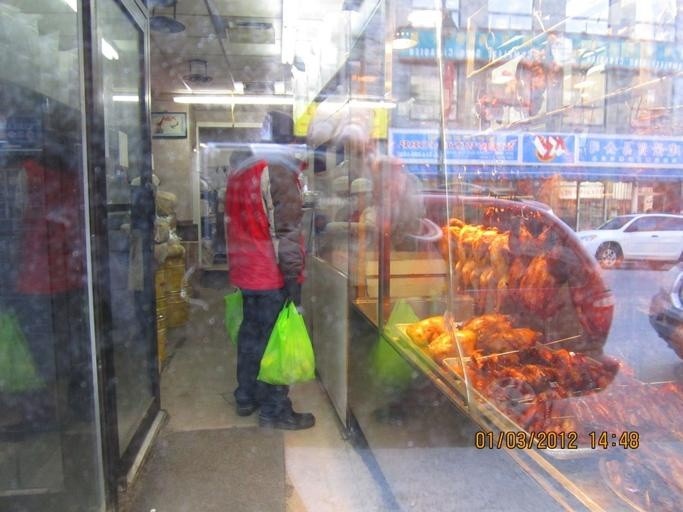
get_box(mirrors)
[196,123,264,272]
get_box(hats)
[259,111,296,143]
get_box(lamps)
[183,59,213,84]
[150,0,186,33]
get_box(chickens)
[406,311,545,367]
[433,215,567,324]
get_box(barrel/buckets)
[155,269,167,364]
[165,257,188,326]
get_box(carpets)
[128,426,287,512]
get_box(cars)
[648,262,682,361]
[575,214,683,269]
[420,190,618,440]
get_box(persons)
[1,112,95,443]
[223,110,316,431]
[3,113,41,169]
[226,146,253,170]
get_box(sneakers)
[236,401,259,416]
[259,410,314,430]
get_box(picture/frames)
[149,112,188,139]
